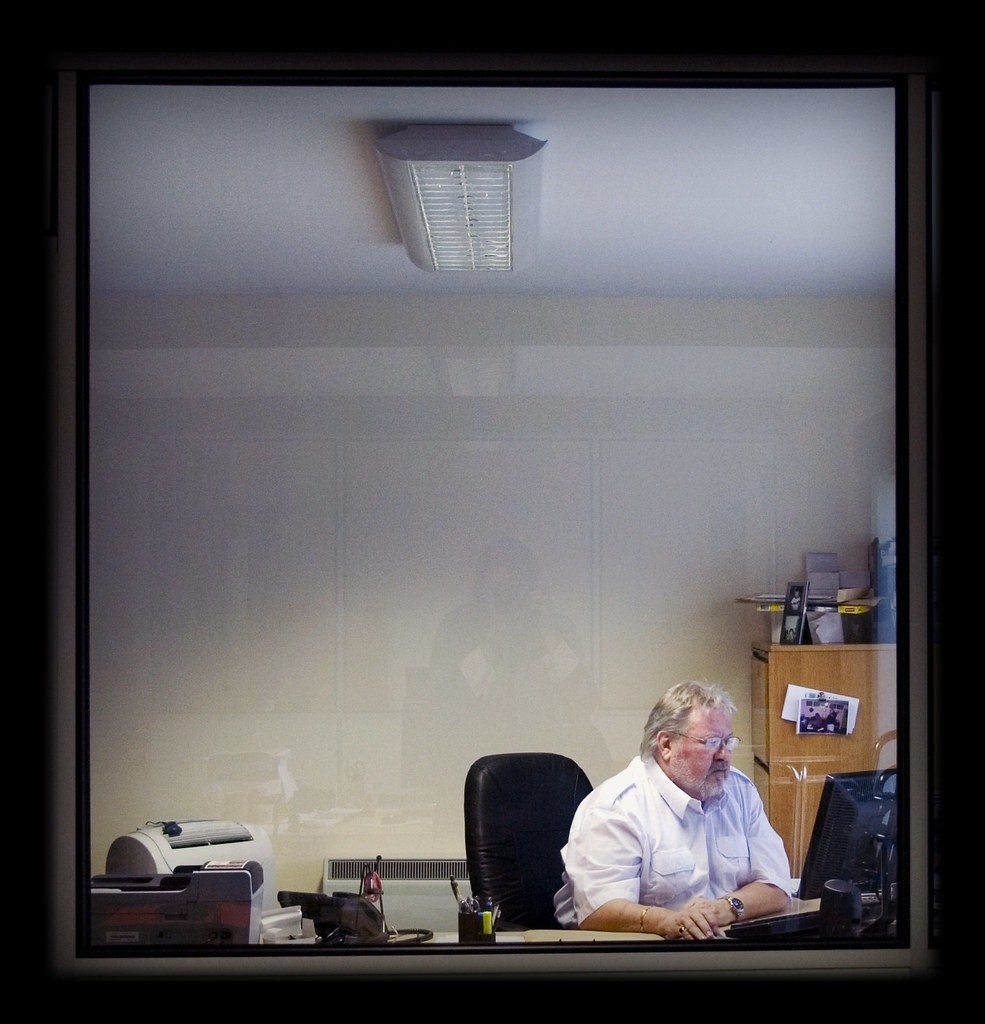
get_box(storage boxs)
[802,552,870,597]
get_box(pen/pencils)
[448,874,501,935]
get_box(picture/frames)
[780,581,810,644]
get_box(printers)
[89,819,279,946]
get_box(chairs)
[464,753,593,932]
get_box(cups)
[458,911,478,944]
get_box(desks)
[419,899,821,943]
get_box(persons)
[801,707,840,733]
[550,681,794,940]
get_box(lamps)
[373,123,547,275]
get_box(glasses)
[669,730,740,752]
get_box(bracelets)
[640,905,655,932]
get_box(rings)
[678,927,686,935]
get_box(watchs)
[718,895,746,922]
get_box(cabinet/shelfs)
[751,643,896,877]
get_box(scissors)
[460,900,480,913]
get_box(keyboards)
[724,898,883,939]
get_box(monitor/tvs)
[796,769,899,933]
